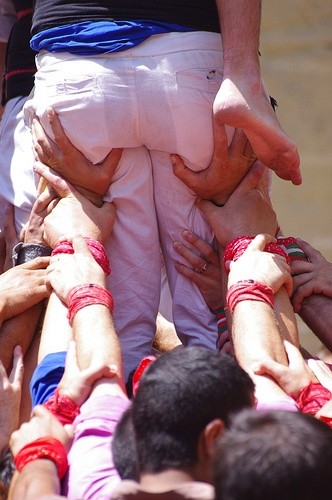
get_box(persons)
[0,0,332,500]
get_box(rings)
[195,263,209,274]
